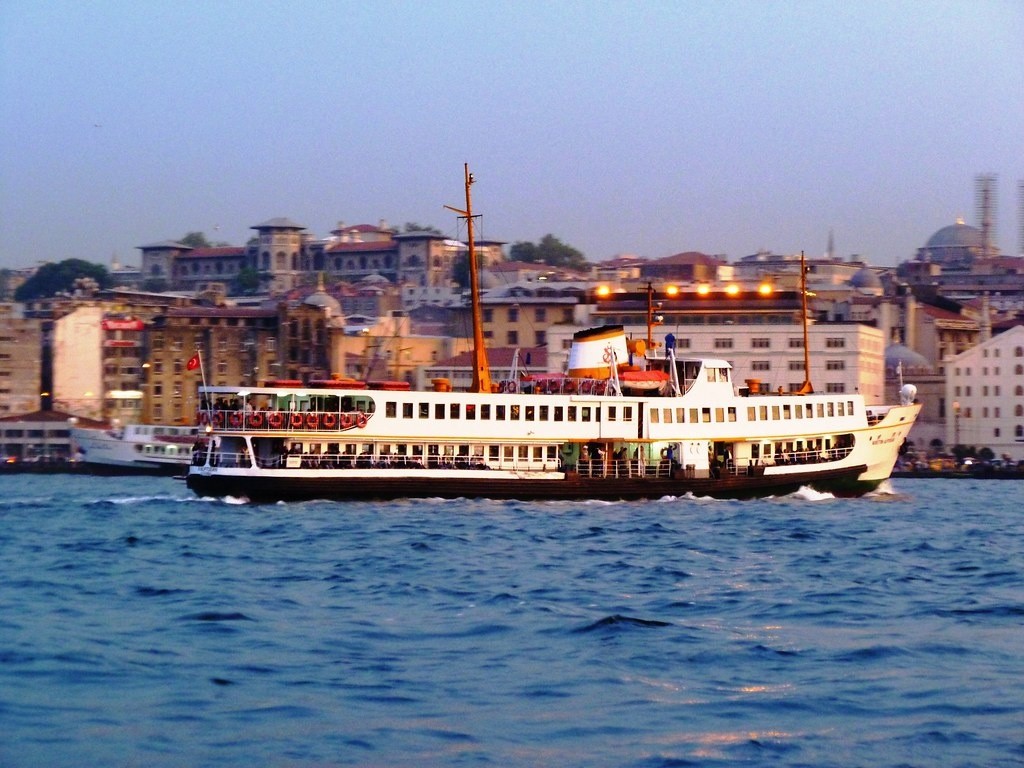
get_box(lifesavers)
[228,412,244,427]
[195,412,209,427]
[305,413,319,429]
[269,413,284,428]
[659,446,671,459]
[289,413,304,428]
[506,378,607,394]
[321,413,337,429]
[355,414,368,429]
[339,414,353,429]
[211,411,224,425]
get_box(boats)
[68,428,201,472]
[182,158,923,498]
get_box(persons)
[320,452,333,469]
[393,453,399,467]
[283,446,288,468]
[471,454,483,468]
[334,451,350,468]
[612,447,627,474]
[201,397,253,411]
[784,447,822,464]
[633,446,645,476]
[724,445,733,469]
[430,453,450,468]
[288,448,294,454]
[302,450,320,468]
[775,445,783,465]
[455,453,469,468]
[708,446,714,464]
[356,451,373,469]
[830,444,839,458]
[192,439,259,468]
[667,444,678,465]
[580,445,605,477]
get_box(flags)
[186,354,202,371]
